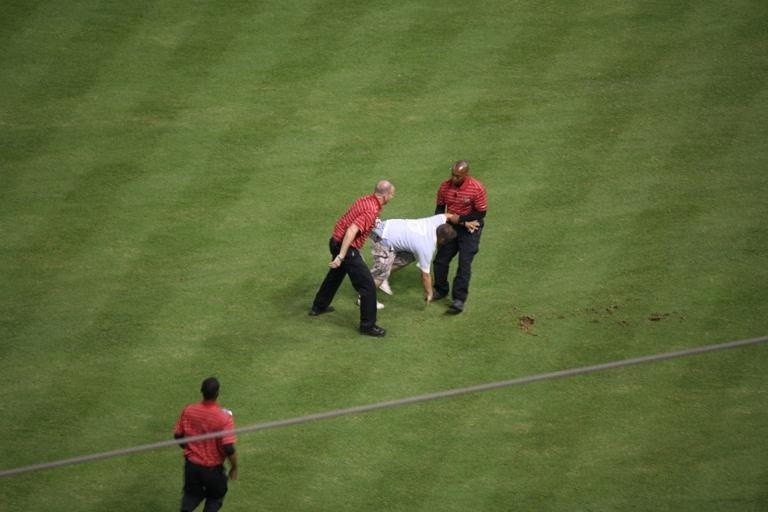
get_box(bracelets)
[335,254,345,264]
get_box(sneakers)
[311,278,464,336]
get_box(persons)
[423,157,489,312]
[308,178,395,338]
[172,376,239,511]
[356,211,479,308]
